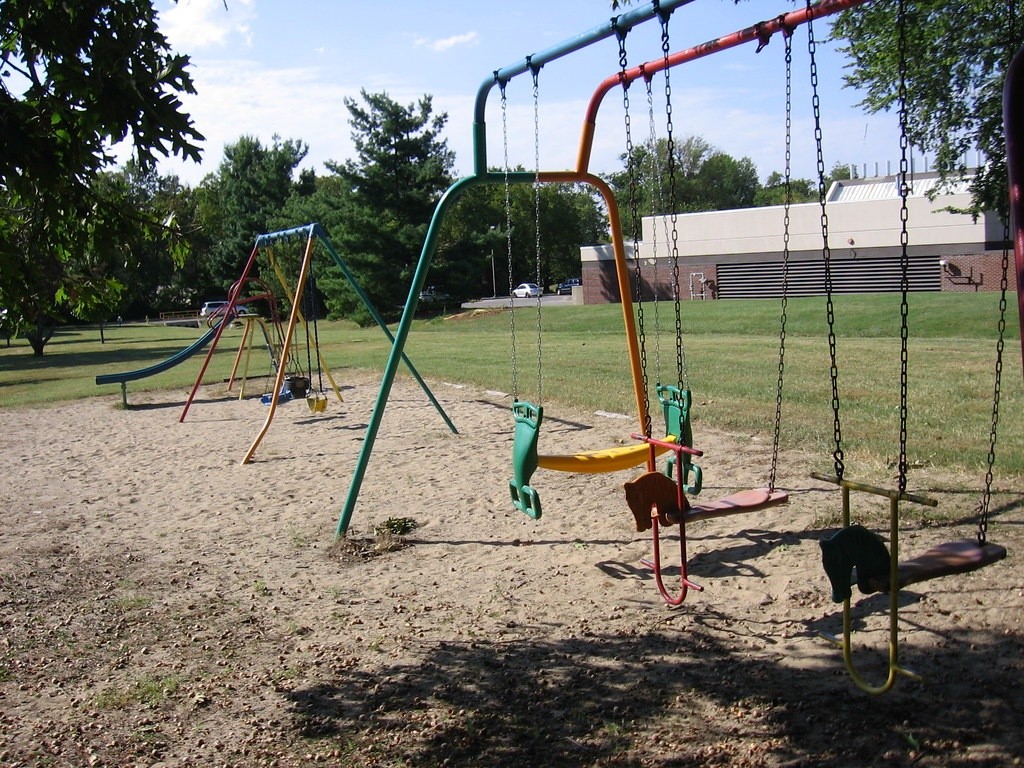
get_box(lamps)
[939,260,949,273]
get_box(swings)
[621,33,803,604]
[260,234,296,409]
[501,25,704,520]
[802,9,1017,695]
[298,231,329,414]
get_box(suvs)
[200,301,248,318]
[555,277,583,296]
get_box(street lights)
[490,225,497,297]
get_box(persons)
[145,315,150,324]
[117,316,122,326]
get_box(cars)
[420,285,463,307]
[511,283,543,298]
